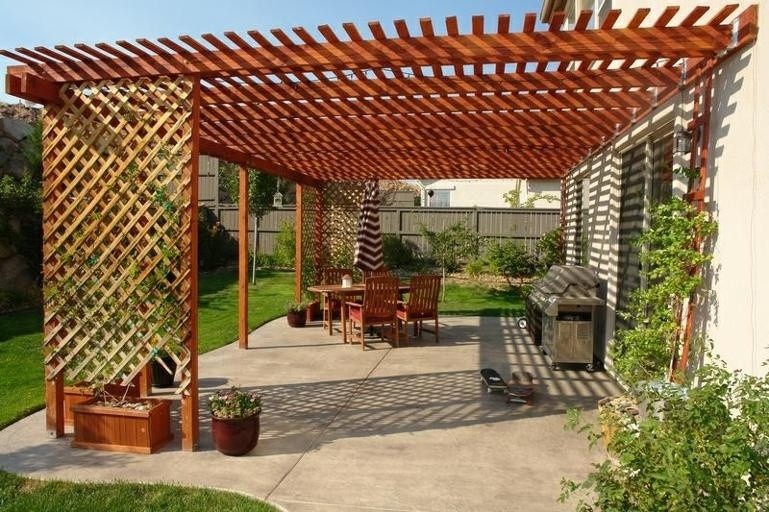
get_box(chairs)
[309,268,442,353]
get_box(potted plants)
[286,301,308,328]
[147,339,182,390]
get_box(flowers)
[209,387,263,420]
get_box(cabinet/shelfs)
[523,298,595,373]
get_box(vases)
[212,421,260,455]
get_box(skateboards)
[505,371,534,406]
[479,368,508,394]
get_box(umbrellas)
[351,180,384,283]
